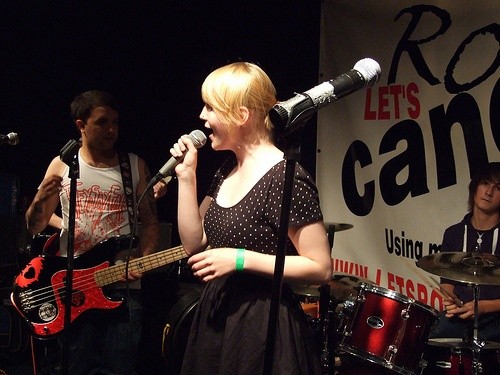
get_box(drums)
[425,337,500,375]
[338,281,443,375]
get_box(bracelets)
[236,248,245,271]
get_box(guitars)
[14,235,211,336]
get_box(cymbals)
[416,251,500,286]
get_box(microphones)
[0,132,19,145]
[270,58,381,127]
[146,130,207,191]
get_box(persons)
[169,62,331,375]
[422,162,500,375]
[25,90,159,375]
